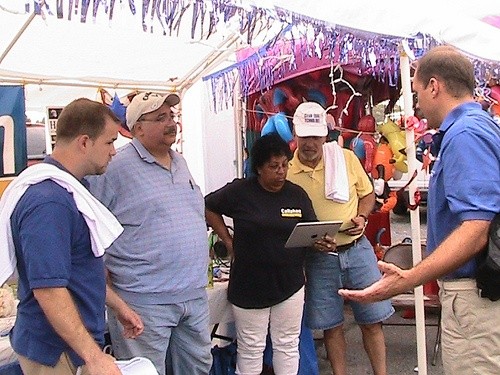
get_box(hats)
[125,91,180,131]
[292,101,328,137]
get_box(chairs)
[376,243,444,367]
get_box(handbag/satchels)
[475,209,500,303]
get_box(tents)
[0,1,425,374]
[232,1,499,182]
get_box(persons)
[8,97,143,375]
[286,102,395,374]
[203,134,338,374]
[338,46,500,375]
[83,92,214,375]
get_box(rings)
[326,244,331,248]
[330,239,336,244]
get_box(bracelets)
[357,214,368,225]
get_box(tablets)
[283,220,343,250]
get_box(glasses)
[136,113,179,122]
